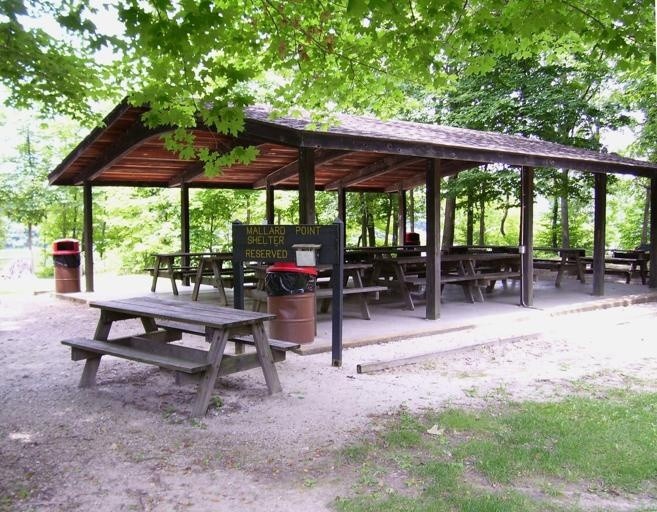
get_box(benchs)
[62,337,213,375]
[533,250,652,286]
[156,320,300,351]
[143,250,519,320]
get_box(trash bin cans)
[405,232,421,256]
[266,262,318,344]
[52,238,81,293]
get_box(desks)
[78,295,282,418]
